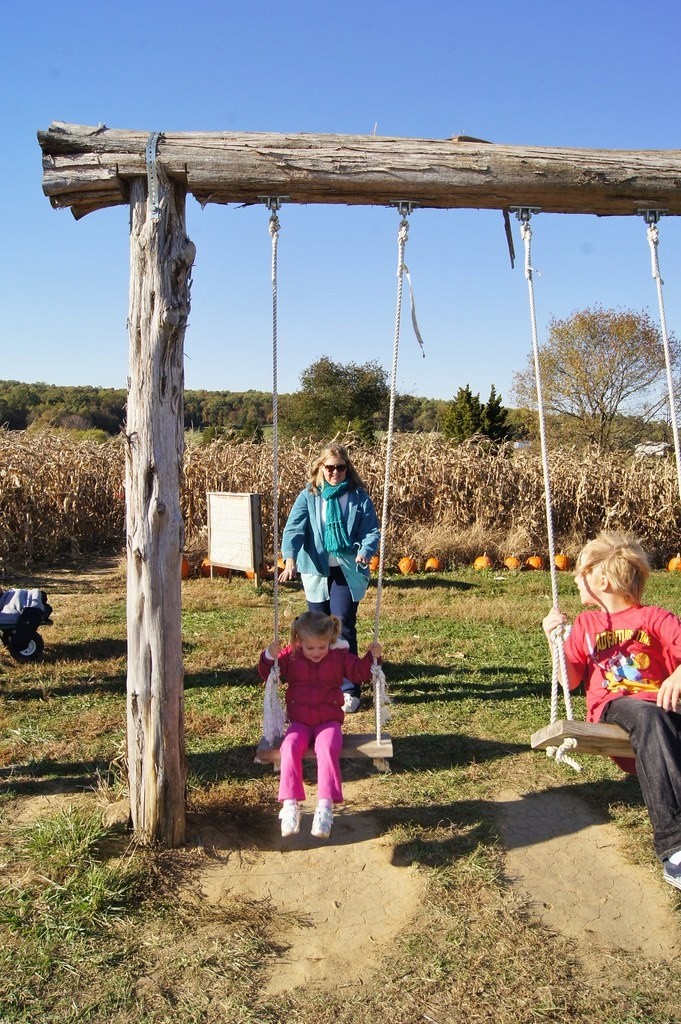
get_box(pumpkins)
[368,550,570,572]
[668,552,681,573]
[181,554,286,580]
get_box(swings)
[509,207,681,758]
[254,195,424,763]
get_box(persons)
[279,444,381,712]
[542,534,681,890]
[257,612,380,839]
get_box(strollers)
[0,586,53,663]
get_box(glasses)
[322,462,348,472]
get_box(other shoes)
[341,692,360,713]
[663,858,681,892]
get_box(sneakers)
[278,804,302,837]
[311,805,334,839]
